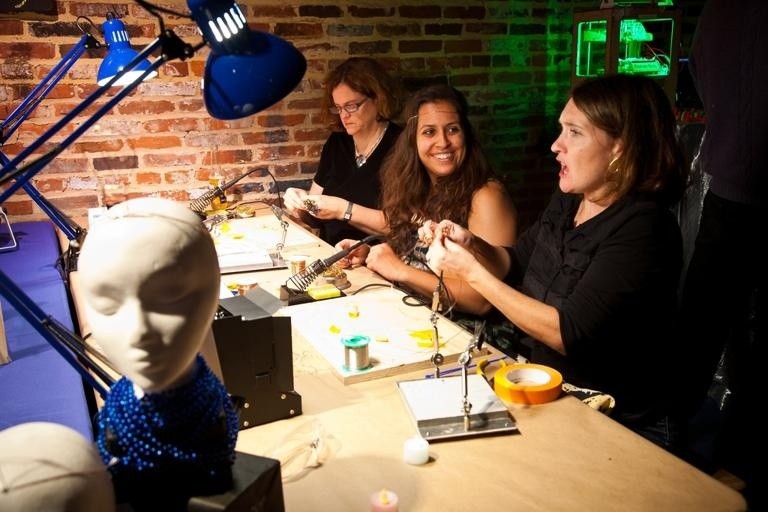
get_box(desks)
[47,208,745,509]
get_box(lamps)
[1,11,162,284]
[2,0,308,400]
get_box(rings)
[426,258,430,265]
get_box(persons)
[74,198,240,502]
[282,56,411,246]
[423,73,701,463]
[332,84,520,317]
[680,0,768,512]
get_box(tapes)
[495,363,563,408]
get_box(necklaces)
[354,126,388,169]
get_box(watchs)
[343,200,353,225]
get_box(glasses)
[330,98,371,114]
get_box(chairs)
[610,188,749,424]
